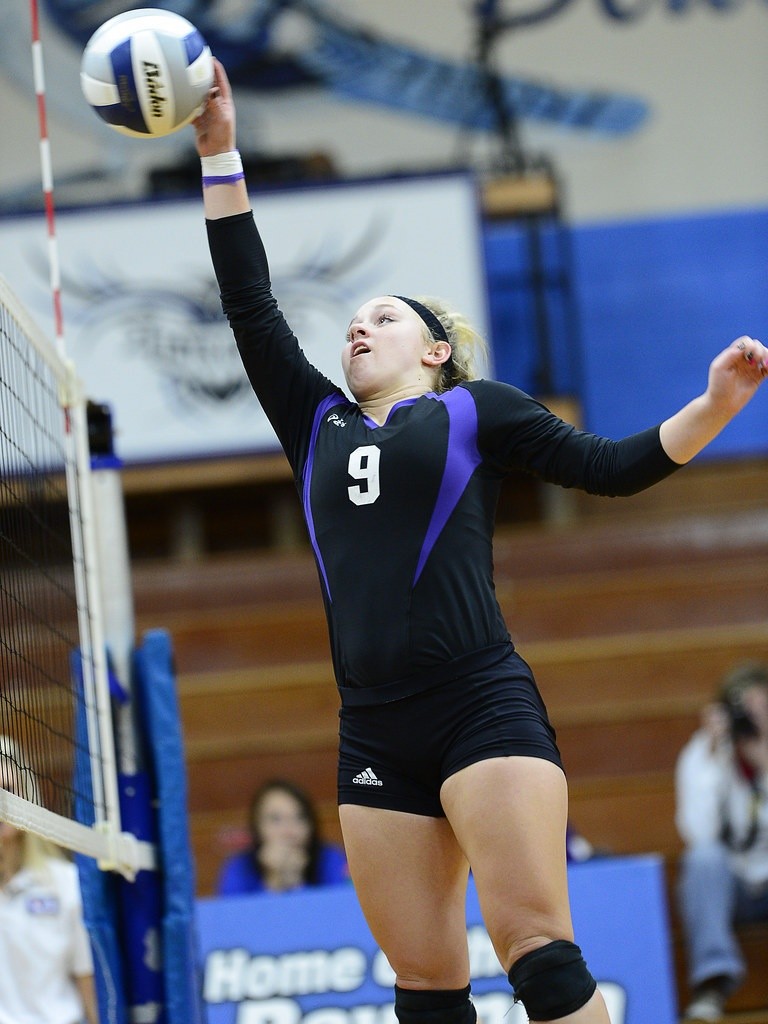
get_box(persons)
[182,43,768,1024]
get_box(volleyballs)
[82,9,216,139]
